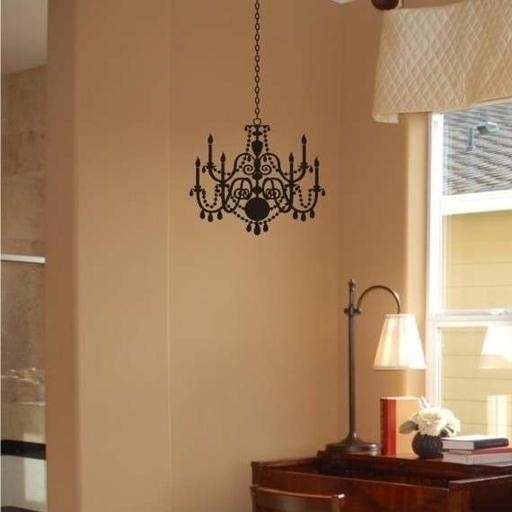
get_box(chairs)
[248,485,345,512]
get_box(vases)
[411,432,443,458]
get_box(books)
[380,396,424,456]
[440,434,512,466]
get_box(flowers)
[397,394,463,437]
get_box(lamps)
[325,278,427,454]
[189,0,326,235]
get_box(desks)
[250,448,512,511]
